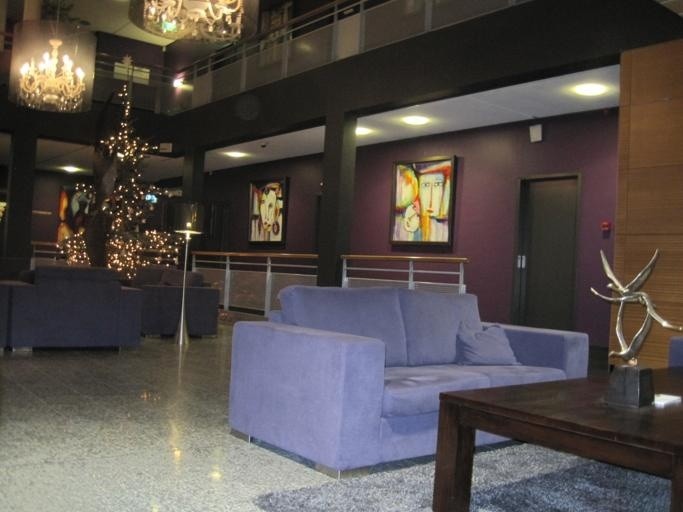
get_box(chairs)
[0,266,145,353]
[130,264,219,339]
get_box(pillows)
[457,321,522,366]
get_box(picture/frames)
[247,176,290,245]
[388,154,457,247]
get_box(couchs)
[228,286,591,480]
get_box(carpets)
[252,442,672,511]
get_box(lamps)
[127,0,260,44]
[173,217,203,344]
[9,0,98,113]
